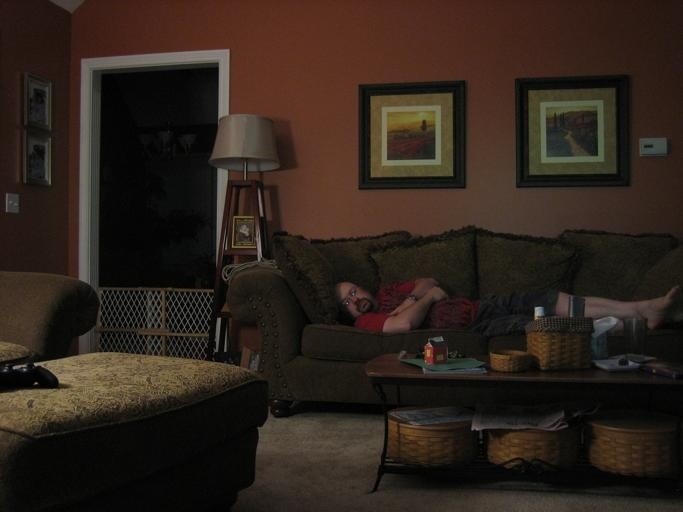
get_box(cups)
[623,317,647,362]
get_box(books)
[640,359,683,379]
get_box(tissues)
[592,315,619,358]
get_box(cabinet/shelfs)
[201,179,270,362]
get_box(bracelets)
[406,294,417,301]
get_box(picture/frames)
[24,72,54,131]
[515,76,623,188]
[358,80,467,189]
[22,129,52,187]
[233,216,254,248]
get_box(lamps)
[209,112,279,177]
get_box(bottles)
[534,306,546,321]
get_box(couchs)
[228,225,683,418]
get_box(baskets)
[585,409,680,479]
[525,316,593,371]
[482,421,577,470]
[386,406,479,464]
[489,349,531,372]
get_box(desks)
[364,352,683,498]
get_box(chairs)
[0,270,101,365]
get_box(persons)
[331,274,681,338]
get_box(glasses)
[341,287,360,306]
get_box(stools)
[0,352,268,511]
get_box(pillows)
[272,222,357,326]
[630,245,682,330]
[475,225,573,304]
[368,224,475,301]
[312,229,409,307]
[561,231,674,296]
